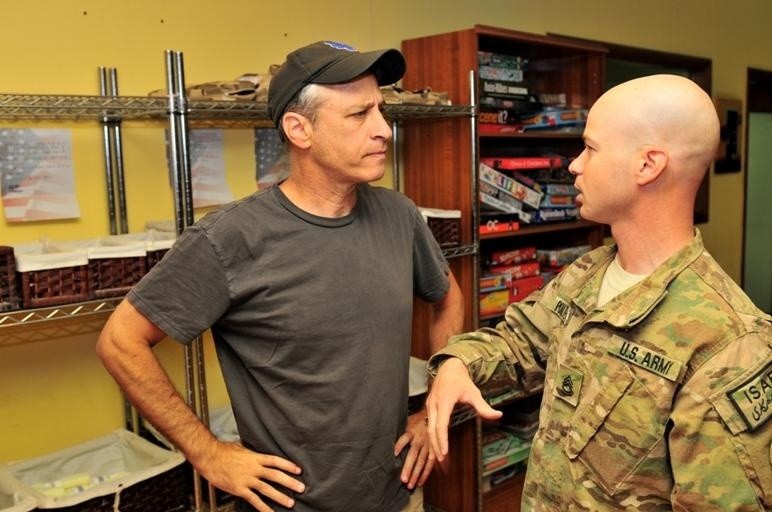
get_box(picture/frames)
[546,29,713,226]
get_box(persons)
[424,73,772,512]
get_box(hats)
[267,40,406,130]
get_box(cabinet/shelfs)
[0,49,206,511]
[401,23,606,511]
